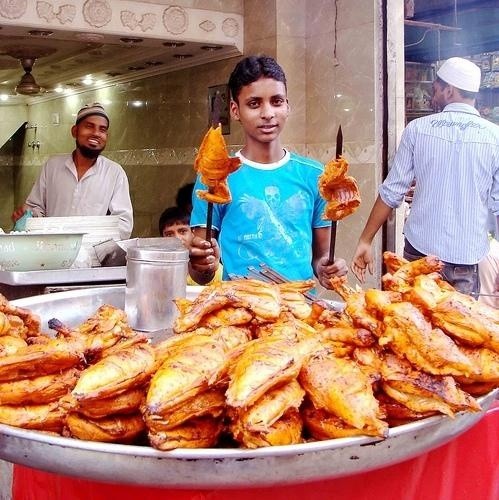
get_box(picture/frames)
[208,83,231,135]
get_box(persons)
[175,182,202,212]
[158,208,224,286]
[351,56,499,300]
[477,236,499,311]
[11,103,134,269]
[187,55,348,299]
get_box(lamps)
[6,48,58,95]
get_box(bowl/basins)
[0,231,87,272]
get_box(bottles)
[124,241,191,334]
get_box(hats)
[436,57,482,92]
[76,102,109,126]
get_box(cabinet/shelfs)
[405,51,498,127]
